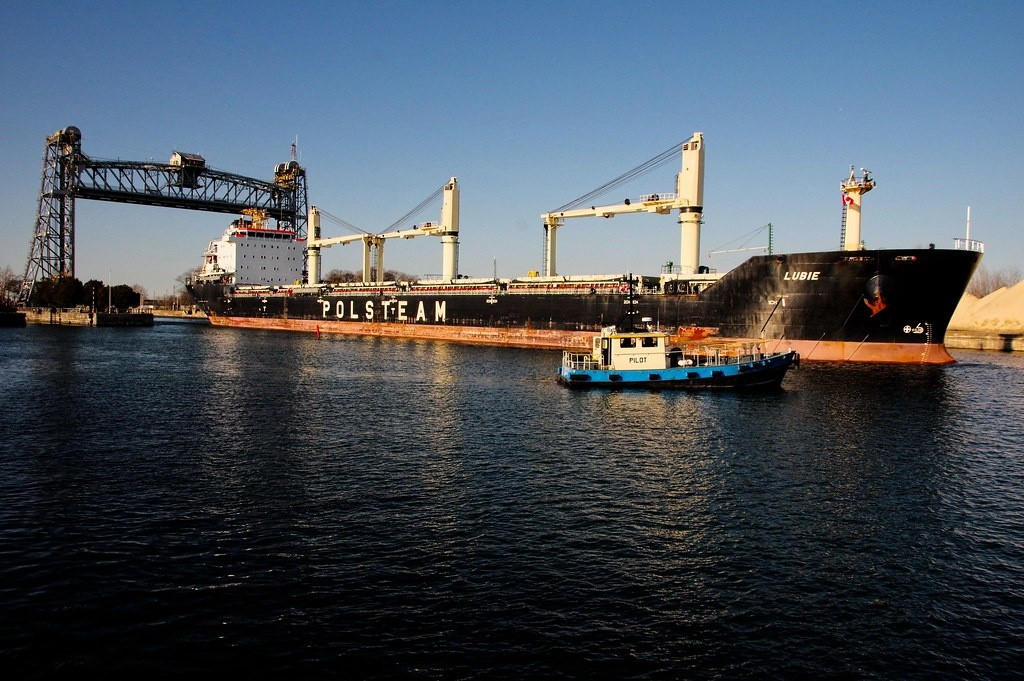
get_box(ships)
[184,131,988,368]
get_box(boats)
[557,272,801,394]
